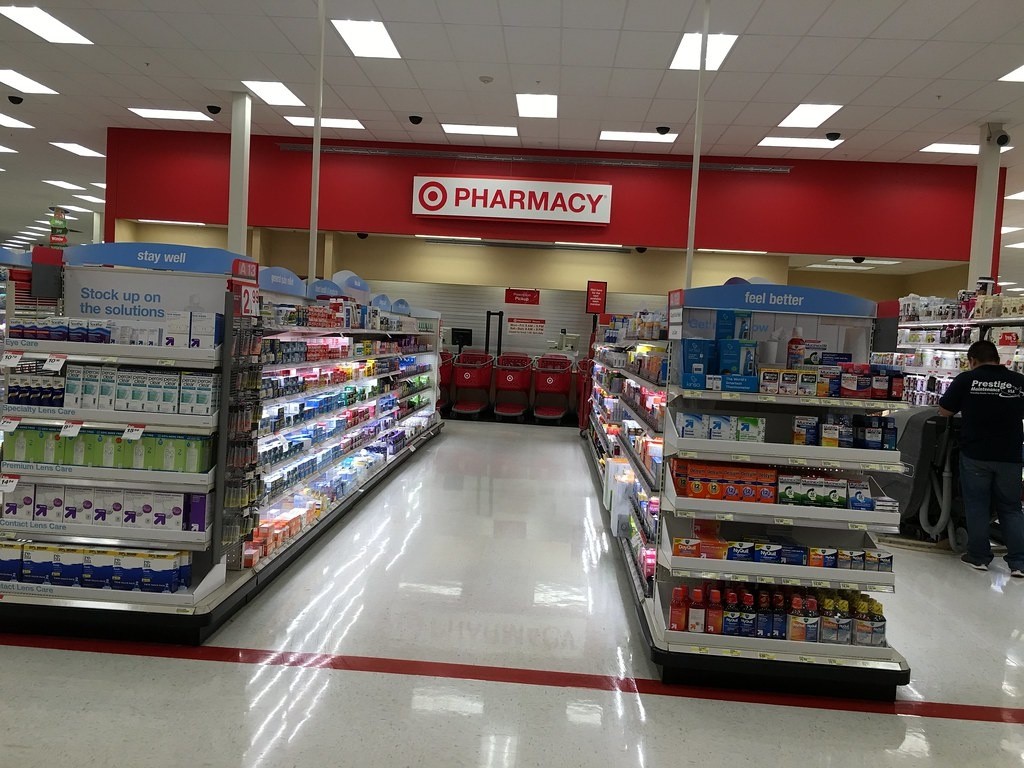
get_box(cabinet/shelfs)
[871,317,1024,379]
[0,268,448,647]
[579,282,910,703]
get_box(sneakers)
[1010,569,1024,577]
[960,552,987,570]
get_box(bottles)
[939,324,972,344]
[786,326,806,371]
[592,366,666,445]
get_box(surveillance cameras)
[825,133,841,141]
[39,243,43,246]
[8,96,23,104]
[409,116,423,125]
[207,106,221,114]
[994,130,1011,147]
[636,247,647,253]
[357,233,368,239]
[852,257,865,263]
[656,127,670,135]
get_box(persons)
[938,340,1024,578]
[799,387,808,394]
[761,385,769,391]
[721,369,731,375]
[780,386,788,393]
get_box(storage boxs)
[2,295,381,593]
[593,336,898,584]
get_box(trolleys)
[531,352,574,427]
[438,350,456,417]
[576,358,592,440]
[449,349,496,421]
[493,351,534,423]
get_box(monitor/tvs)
[557,333,580,351]
[440,327,472,347]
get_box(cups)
[758,340,778,364]
